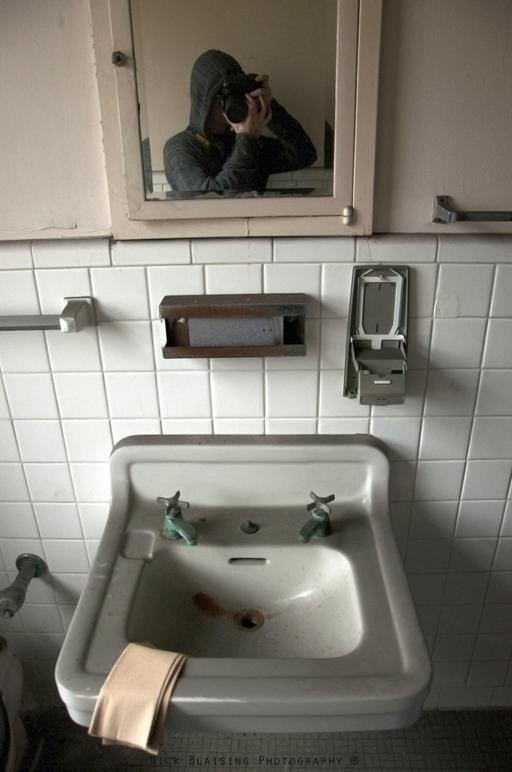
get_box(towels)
[86,638,189,761]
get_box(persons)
[161,47,318,194]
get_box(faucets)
[297,493,334,543]
[156,490,198,548]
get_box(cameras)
[217,72,262,124]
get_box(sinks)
[54,435,432,728]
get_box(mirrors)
[108,0,360,224]
